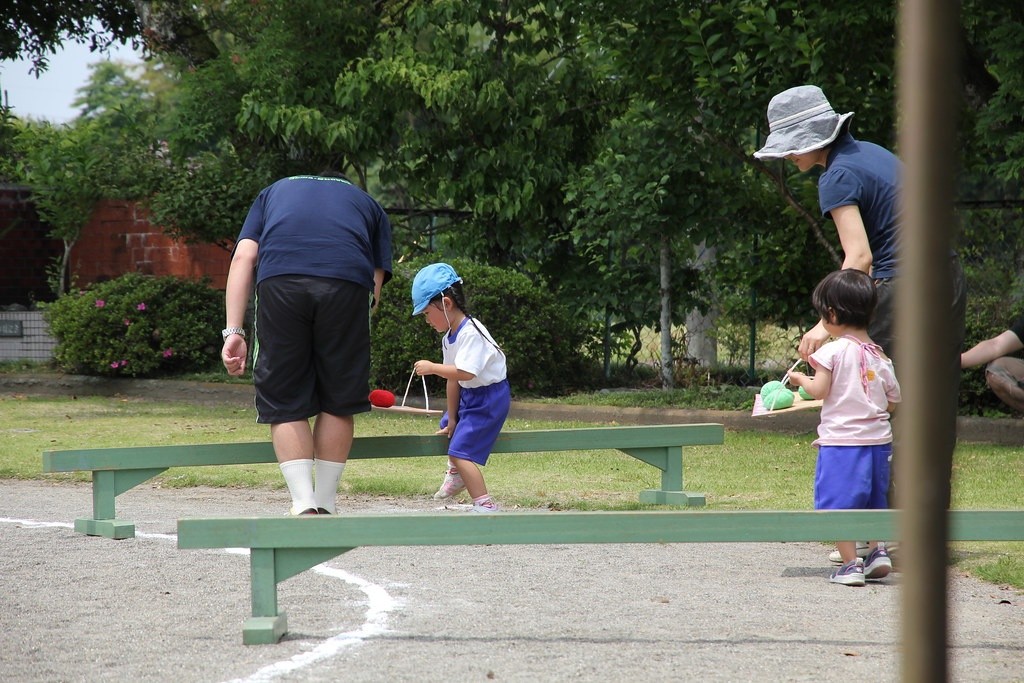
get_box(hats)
[753,85,855,158]
[412,263,462,315]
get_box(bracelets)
[221,327,246,342]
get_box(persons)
[220,169,394,516]
[411,262,512,512]
[960,314,1024,414]
[751,83,973,567]
[784,265,898,587]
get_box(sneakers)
[434,468,467,500]
[864,542,891,577]
[473,497,498,510]
[830,541,900,561]
[829,558,865,586]
